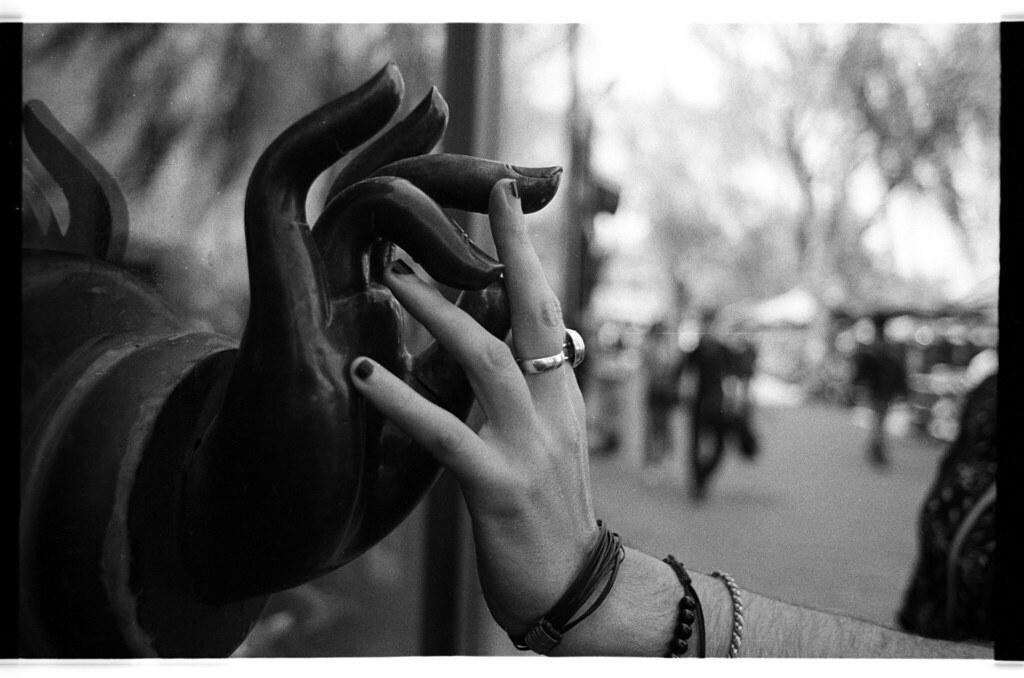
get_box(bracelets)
[506,517,744,657]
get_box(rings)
[520,326,585,377]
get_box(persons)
[347,178,999,657]
[0,61,562,655]
[582,308,911,496]
